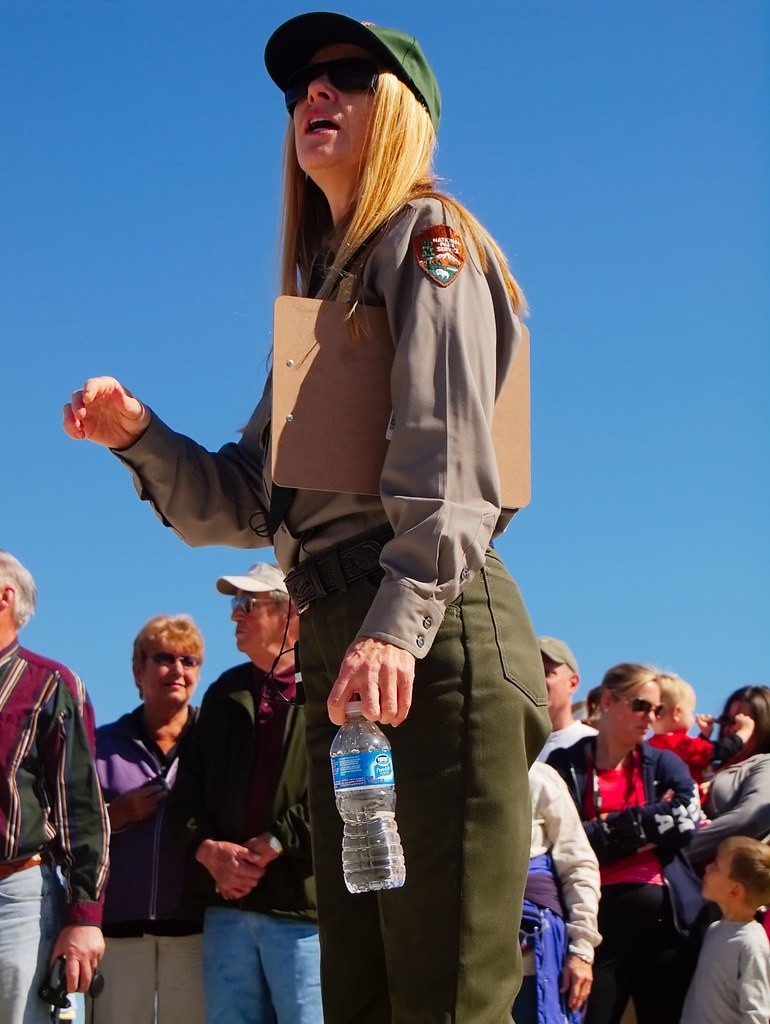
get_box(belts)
[0,853,48,880]
[284,530,395,613]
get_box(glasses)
[231,597,277,611]
[286,52,379,119]
[608,687,663,717]
[140,653,201,667]
[708,714,737,726]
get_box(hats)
[537,634,579,676]
[264,12,440,125]
[217,562,290,595]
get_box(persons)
[514,635,770,1024]
[63,11,552,1024]
[84,614,209,1024]
[0,550,111,1024]
[163,561,324,1024]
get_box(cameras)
[39,954,105,1008]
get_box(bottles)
[330,701,405,892]
